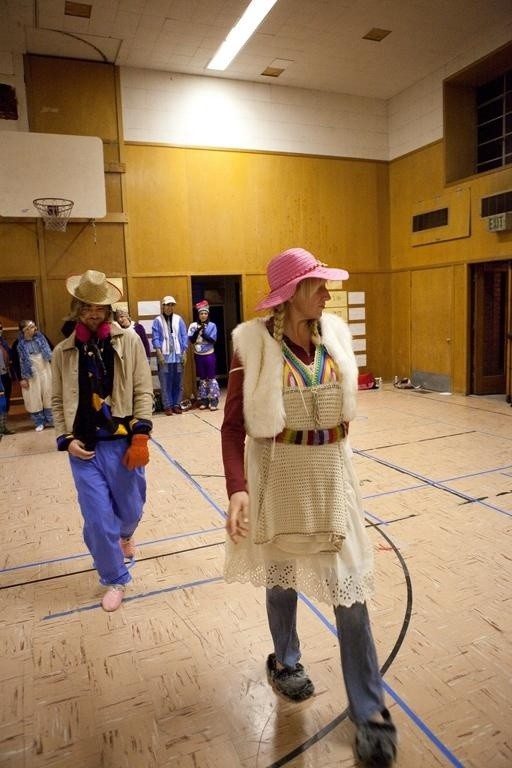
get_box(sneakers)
[208,403,216,410]
[174,408,181,414]
[200,405,207,409]
[165,409,172,415]
[35,425,44,431]
[119,538,136,558]
[102,585,125,612]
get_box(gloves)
[122,434,150,471]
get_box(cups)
[374,378,379,387]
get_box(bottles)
[379,376,384,387]
[190,393,195,402]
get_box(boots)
[0,414,15,434]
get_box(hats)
[65,270,123,306]
[162,296,176,305]
[256,248,350,311]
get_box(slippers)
[346,704,396,767]
[266,653,313,699]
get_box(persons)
[0,296,217,435]
[221,249,398,768]
[49,269,155,612]
